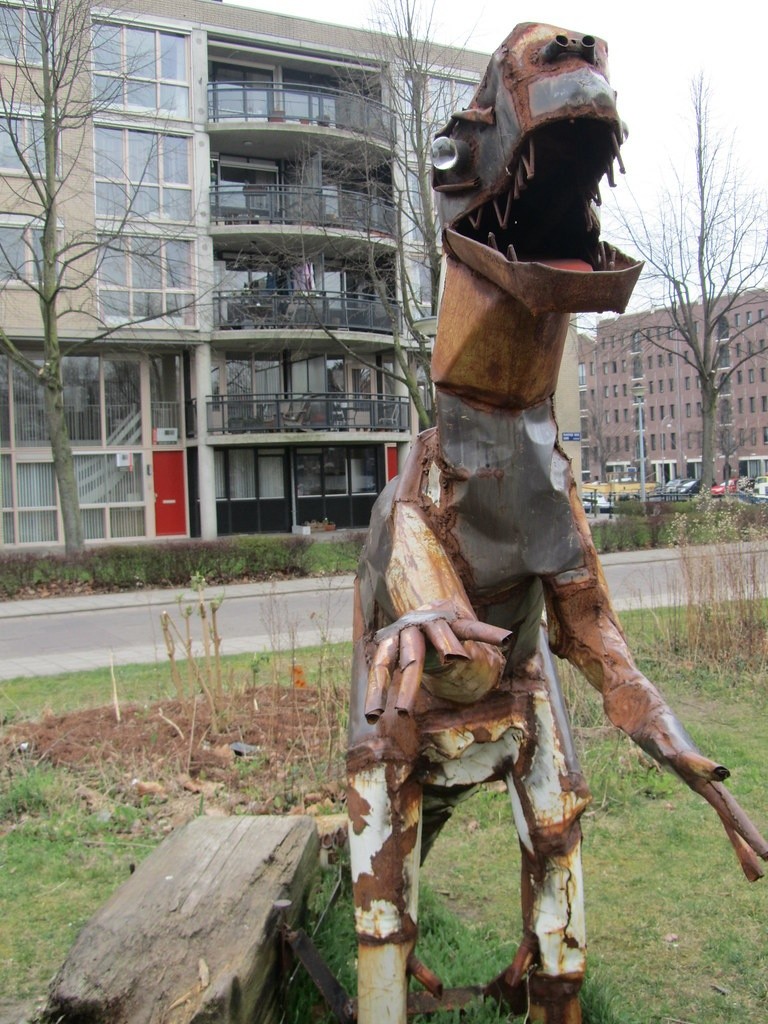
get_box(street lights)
[631,377,647,516]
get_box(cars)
[752,476,768,498]
[582,497,615,506]
[711,478,740,494]
[656,479,689,500]
[583,504,626,527]
[670,480,700,500]
[605,491,641,502]
[581,491,604,498]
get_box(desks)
[338,407,361,431]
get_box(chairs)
[378,403,399,432]
[281,393,322,432]
